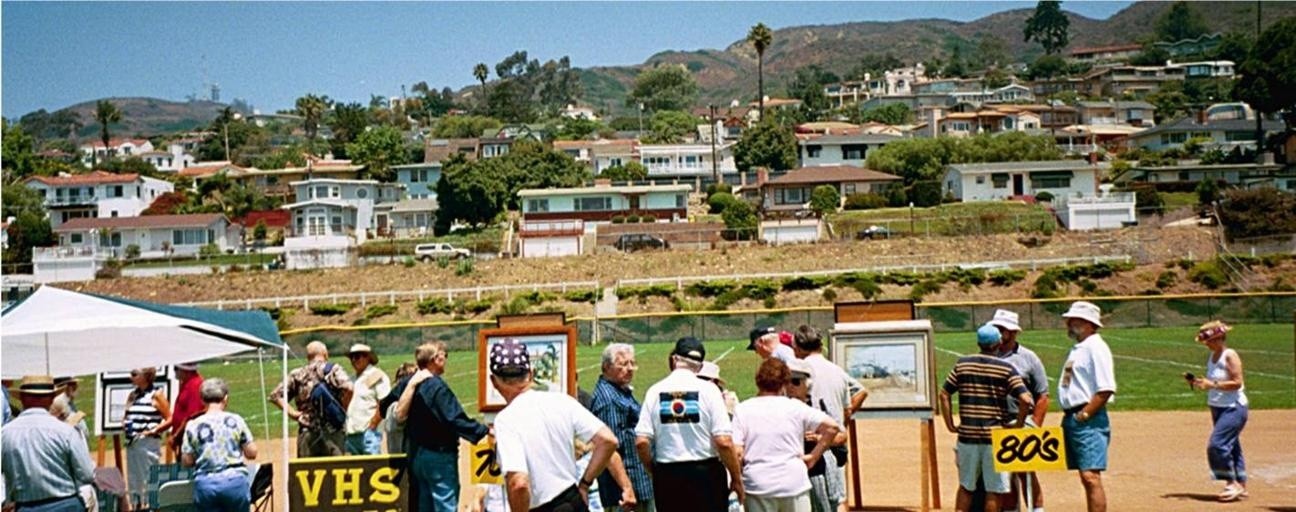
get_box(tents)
[1,286,289,512]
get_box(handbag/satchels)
[310,379,346,427]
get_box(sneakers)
[1219,483,1248,501]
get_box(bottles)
[362,425,382,455]
[728,490,741,512]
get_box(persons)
[634,336,746,512]
[379,340,495,512]
[939,325,1031,512]
[270,341,391,459]
[181,378,258,512]
[576,373,591,409]
[986,309,1050,512]
[489,342,618,512]
[732,358,839,512]
[590,344,654,512]
[122,367,173,512]
[746,326,867,512]
[168,362,208,462]
[1057,302,1117,512]
[1182,320,1250,502]
[0,375,97,512]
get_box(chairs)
[156,480,198,512]
[249,463,274,512]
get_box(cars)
[415,241,469,263]
[611,233,664,251]
[858,224,892,239]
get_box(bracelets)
[1078,408,1090,421]
[1214,379,1219,390]
[582,479,592,486]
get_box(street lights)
[726,99,738,117]
[637,101,644,133]
[706,100,716,179]
[223,112,241,161]
[1109,97,1119,123]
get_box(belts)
[959,436,991,444]
[1064,404,1087,414]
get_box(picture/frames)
[834,299,915,322]
[478,325,577,413]
[827,326,939,414]
[100,365,168,380]
[101,381,170,431]
[496,311,572,328]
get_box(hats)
[1195,320,1232,342]
[490,344,530,375]
[675,337,705,361]
[7,376,68,399]
[786,359,810,378]
[346,344,378,364]
[988,309,1021,331]
[747,326,776,349]
[1062,302,1104,328]
[697,361,727,385]
[977,326,1002,344]
